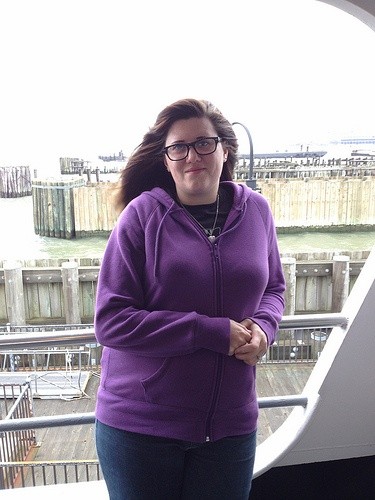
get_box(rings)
[256,355,260,360]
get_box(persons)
[95,99,285,500]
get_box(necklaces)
[177,192,220,243]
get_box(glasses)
[162,136,223,161]
